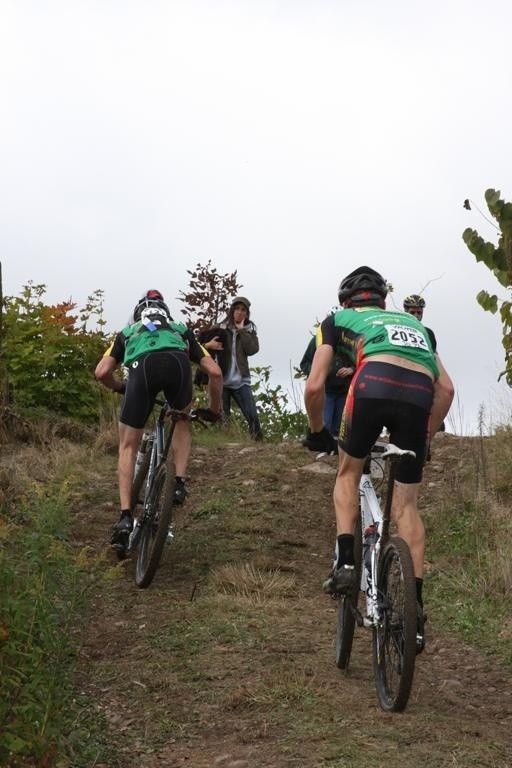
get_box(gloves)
[304,426,334,456]
[111,384,127,395]
[190,407,217,423]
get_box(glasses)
[407,310,424,316]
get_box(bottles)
[365,528,376,571]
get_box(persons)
[199,297,265,442]
[403,294,426,320]
[303,267,454,654]
[94,289,223,550]
[300,336,356,436]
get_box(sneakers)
[171,488,186,505]
[393,632,425,652]
[322,563,359,595]
[108,517,134,539]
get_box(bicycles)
[303,434,433,712]
[105,388,217,589]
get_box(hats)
[230,296,251,309]
[336,266,388,305]
[403,294,426,308]
[132,296,175,322]
[146,289,161,298]
